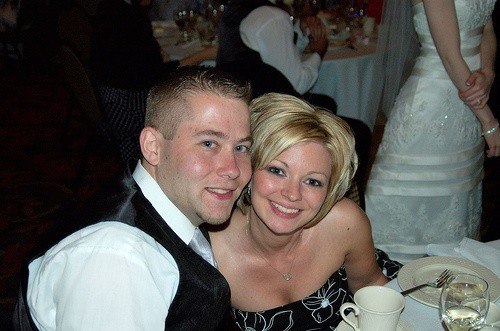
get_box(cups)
[363,17,375,35]
[339,285,406,331]
[173,0,228,47]
[439,273,490,331]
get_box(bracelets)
[482,119,499,136]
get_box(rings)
[476,97,481,104]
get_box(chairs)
[54,3,132,126]
[54,38,121,206]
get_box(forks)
[400,269,454,296]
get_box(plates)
[397,256,500,310]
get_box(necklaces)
[246,204,303,281]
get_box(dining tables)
[149,10,386,134]
[381,240,500,331]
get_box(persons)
[0,0,500,331]
[11,65,253,331]
[208,92,389,331]
